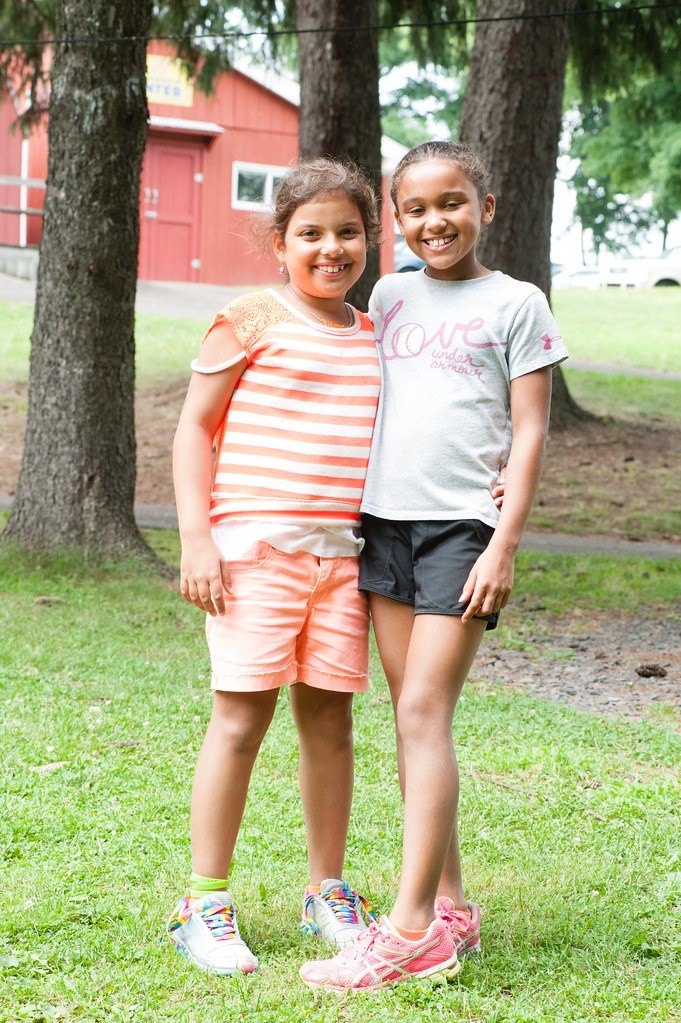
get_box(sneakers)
[299,914,462,994]
[302,878,376,954]
[434,896,481,957]
[166,890,258,975]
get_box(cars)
[643,244,681,286]
[393,240,564,278]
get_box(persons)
[165,156,504,975]
[298,141,570,992]
